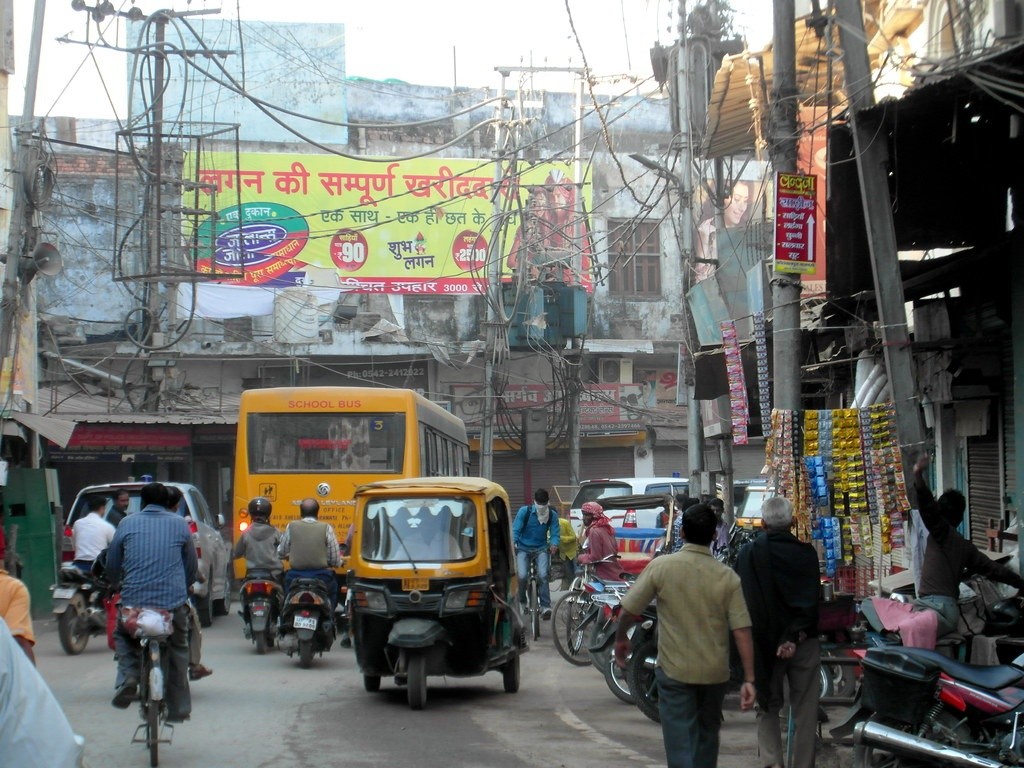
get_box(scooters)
[287,577,348,668]
[240,570,289,655]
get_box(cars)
[61,474,236,628]
[734,480,782,532]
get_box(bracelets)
[744,680,754,685]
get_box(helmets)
[248,498,272,518]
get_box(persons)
[629,380,656,419]
[0,617,85,768]
[614,504,755,768]
[546,506,584,592]
[656,494,730,560]
[392,509,462,561]
[276,498,342,611]
[512,487,560,621]
[861,453,1024,643]
[0,524,6,570]
[231,497,284,617]
[71,496,116,572]
[506,169,591,281]
[0,568,36,667]
[105,483,214,720]
[106,489,129,528]
[341,523,354,648]
[576,502,626,582]
[735,498,821,768]
[693,177,754,283]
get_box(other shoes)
[540,605,552,622]
[110,678,137,711]
[518,600,527,617]
[189,664,214,680]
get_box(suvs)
[567,471,722,540]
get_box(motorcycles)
[849,639,1024,766]
[51,560,111,655]
[586,561,1024,768]
[342,475,529,707]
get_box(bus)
[233,384,472,587]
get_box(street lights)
[628,152,703,504]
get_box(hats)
[534,488,549,505]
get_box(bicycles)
[515,544,559,641]
[121,613,177,768]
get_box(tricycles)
[555,484,682,665]
